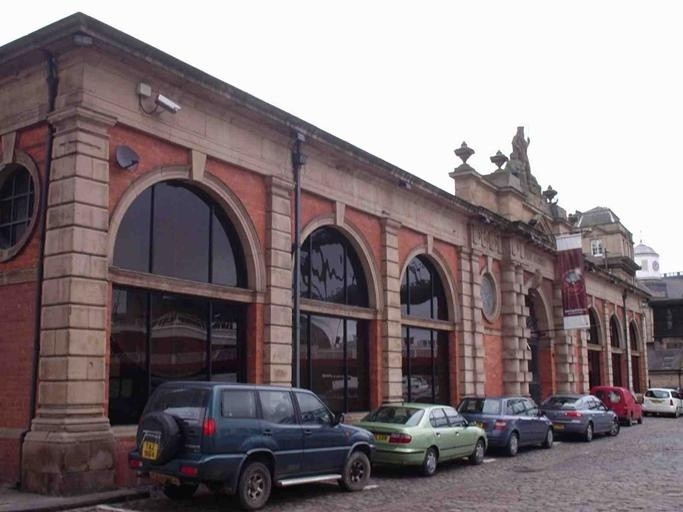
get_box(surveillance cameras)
[154,92,182,115]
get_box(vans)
[590,386,643,425]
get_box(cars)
[456,396,555,453]
[326,362,428,398]
[641,387,682,417]
[355,399,489,472]
[122,381,376,511]
[540,395,618,440]
